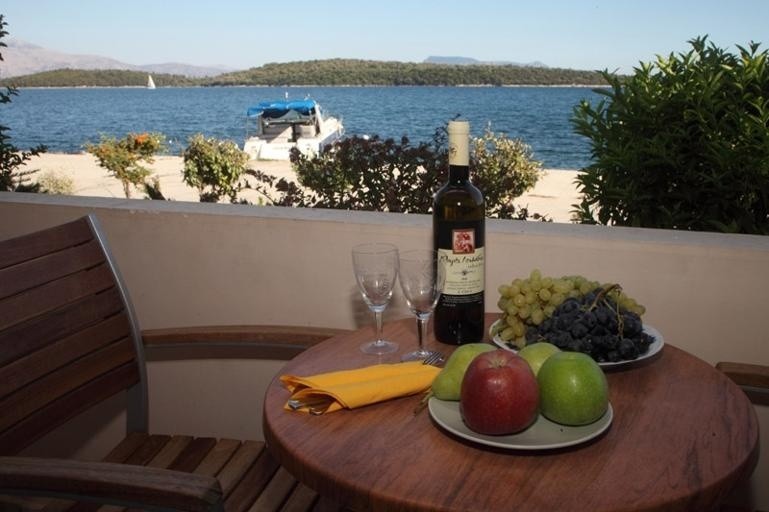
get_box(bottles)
[433,119,486,346]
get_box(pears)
[517,341,561,377]
[413,342,498,416]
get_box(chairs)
[0,213,351,512]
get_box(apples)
[459,350,540,437]
[535,352,609,426]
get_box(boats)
[243,92,346,161]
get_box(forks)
[308,350,444,416]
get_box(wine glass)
[397,248,450,367]
[351,242,402,356]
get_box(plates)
[425,386,616,451]
[488,314,666,371]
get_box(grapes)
[489,269,653,362]
